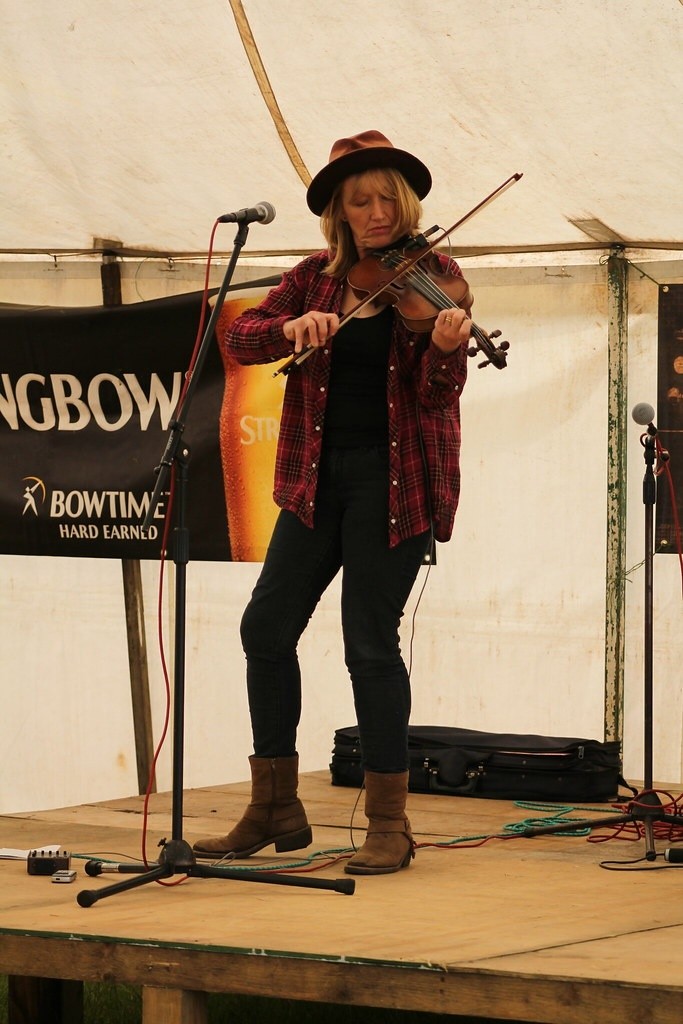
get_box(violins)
[348,233,508,370]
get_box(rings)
[445,316,453,323]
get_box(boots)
[193,753,313,859]
[343,770,418,875]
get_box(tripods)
[76,226,357,907]
[523,438,683,861]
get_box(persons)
[191,130,473,874]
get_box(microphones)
[218,202,274,227]
[633,403,669,460]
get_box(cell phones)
[52,870,77,885]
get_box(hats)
[305,130,432,219]
